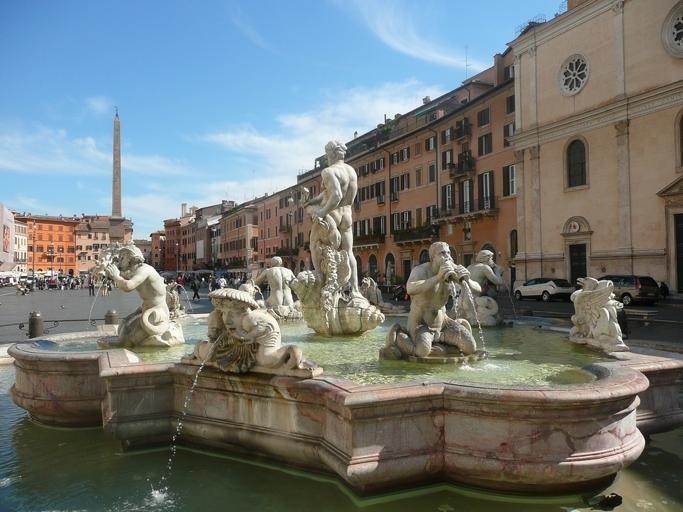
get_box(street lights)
[287,197,294,250]
[58,248,63,273]
[211,225,216,269]
[48,242,54,277]
[159,247,161,267]
[175,243,179,277]
[31,222,37,291]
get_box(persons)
[16,276,117,296]
[208,288,259,333]
[304,139,367,300]
[107,245,169,344]
[164,272,242,302]
[456,250,504,316]
[396,242,477,357]
[254,256,297,305]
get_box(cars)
[19,272,32,286]
[513,278,577,302]
[597,275,660,306]
